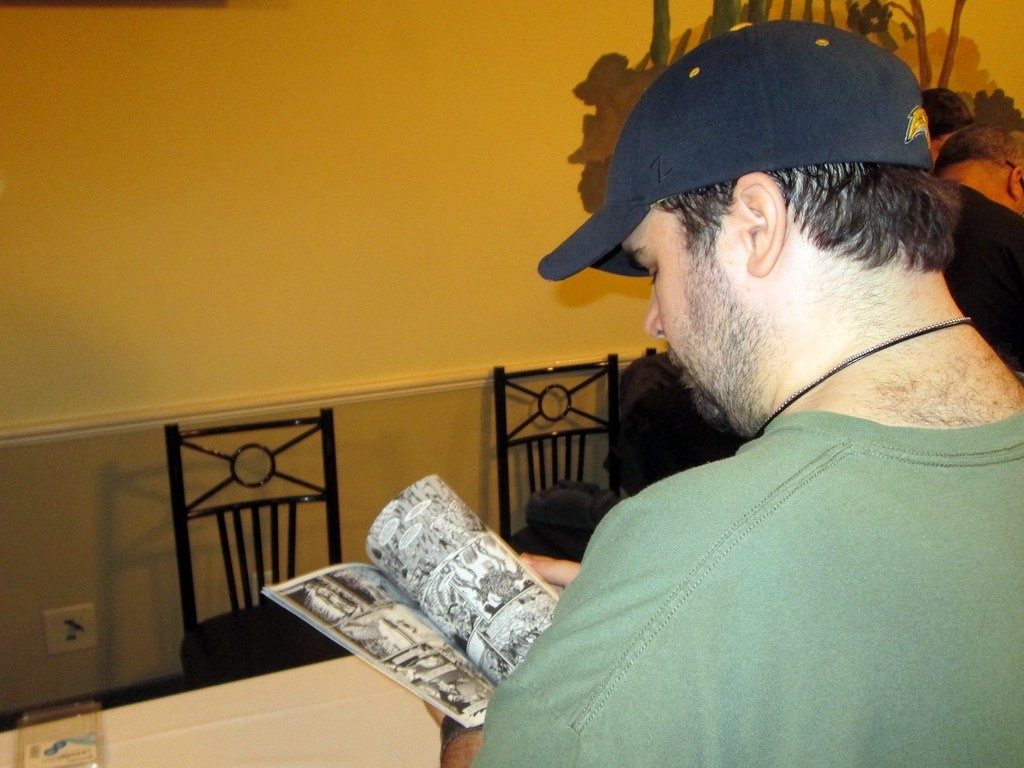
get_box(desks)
[0,651,442,768]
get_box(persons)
[916,87,973,172]
[435,19,1019,767]
[922,126,1024,380]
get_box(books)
[258,473,562,732]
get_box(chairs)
[166,407,358,695]
[495,353,619,562]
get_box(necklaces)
[753,308,986,431]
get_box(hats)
[538,19,933,282]
